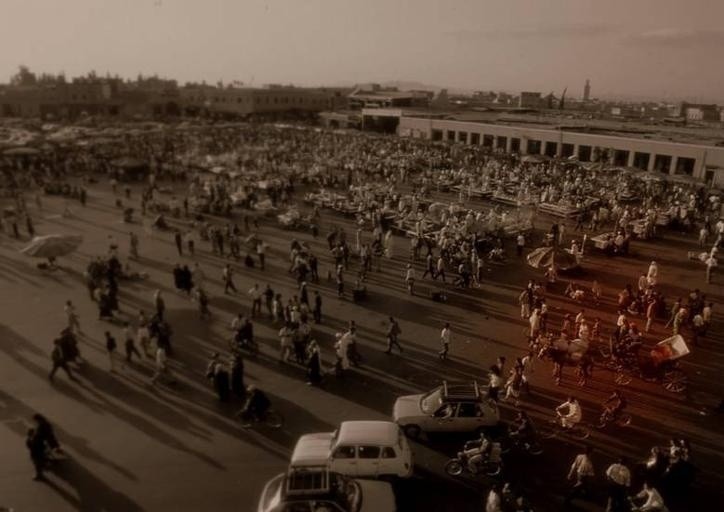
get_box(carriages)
[533,334,693,393]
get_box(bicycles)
[235,406,285,428]
[502,403,632,454]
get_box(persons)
[664,287,714,339]
[400,184,511,294]
[505,150,694,253]
[159,126,342,236]
[463,357,690,510]
[285,226,393,300]
[335,132,512,232]
[383,316,404,355]
[24,413,61,482]
[47,325,82,385]
[1,154,87,239]
[695,184,723,285]
[103,329,117,371]
[172,223,268,319]
[228,280,355,379]
[83,234,126,321]
[515,222,590,287]
[204,345,273,451]
[123,289,176,384]
[518,278,602,386]
[438,323,451,363]
[64,299,83,334]
[77,135,189,229]
[607,258,663,355]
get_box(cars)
[257,380,501,512]
